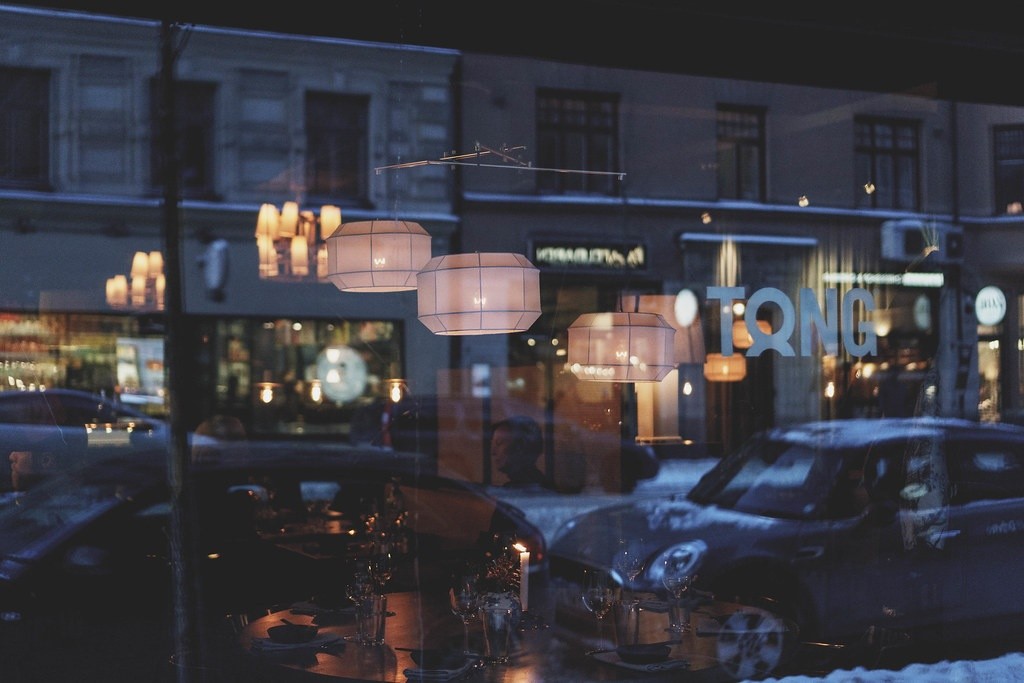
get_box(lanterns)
[567,311,676,384]
[317,219,431,293]
[411,251,541,337]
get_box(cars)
[0,442,550,683]
[542,419,1024,683]
[0,388,220,495]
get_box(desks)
[255,509,402,565]
[239,589,541,683]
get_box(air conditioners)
[928,222,966,267]
[880,220,929,263]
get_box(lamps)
[101,201,679,386]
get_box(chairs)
[218,604,283,644]
[166,649,229,682]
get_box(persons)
[491,416,544,488]
